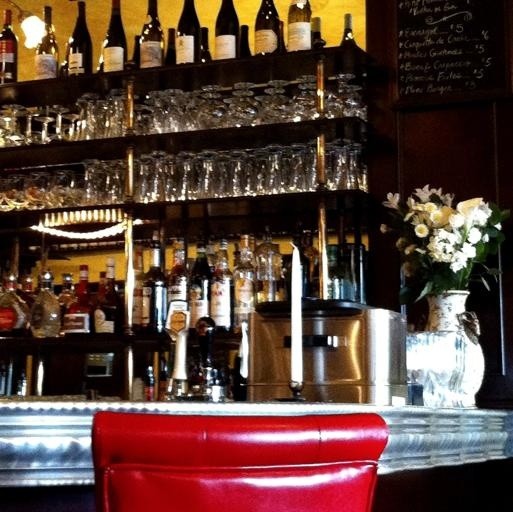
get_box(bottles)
[0,0,357,88]
[1,218,369,341]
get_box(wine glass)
[409,331,467,410]
[1,71,366,147]
[1,137,369,213]
[250,108,253,109]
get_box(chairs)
[91,410,390,512]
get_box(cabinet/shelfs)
[0,43,373,398]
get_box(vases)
[419,288,487,415]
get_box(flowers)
[377,176,511,314]
